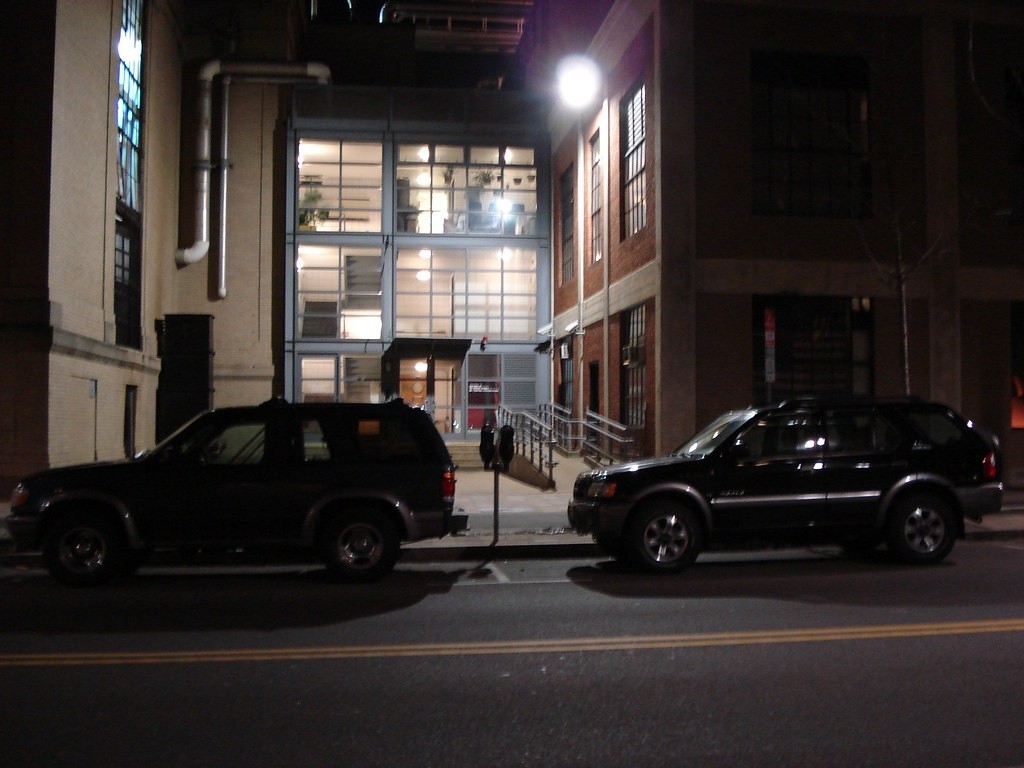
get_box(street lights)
[557,55,602,458]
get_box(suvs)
[567,393,1007,574]
[1,398,471,589]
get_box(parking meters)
[479,424,515,541]
